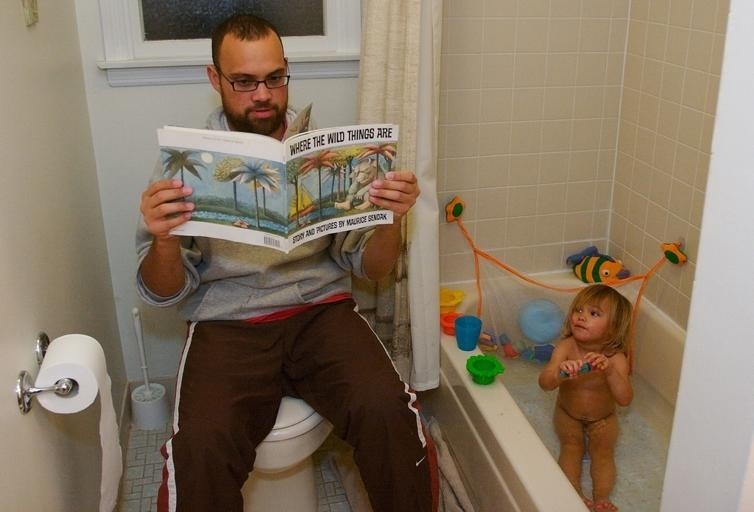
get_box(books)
[156,124,399,253]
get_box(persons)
[131,14,441,512]
[539,284,634,512]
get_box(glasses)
[214,63,290,92]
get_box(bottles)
[560,361,597,378]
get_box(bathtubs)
[421,265,687,512]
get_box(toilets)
[243,389,334,512]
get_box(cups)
[455,314,481,351]
